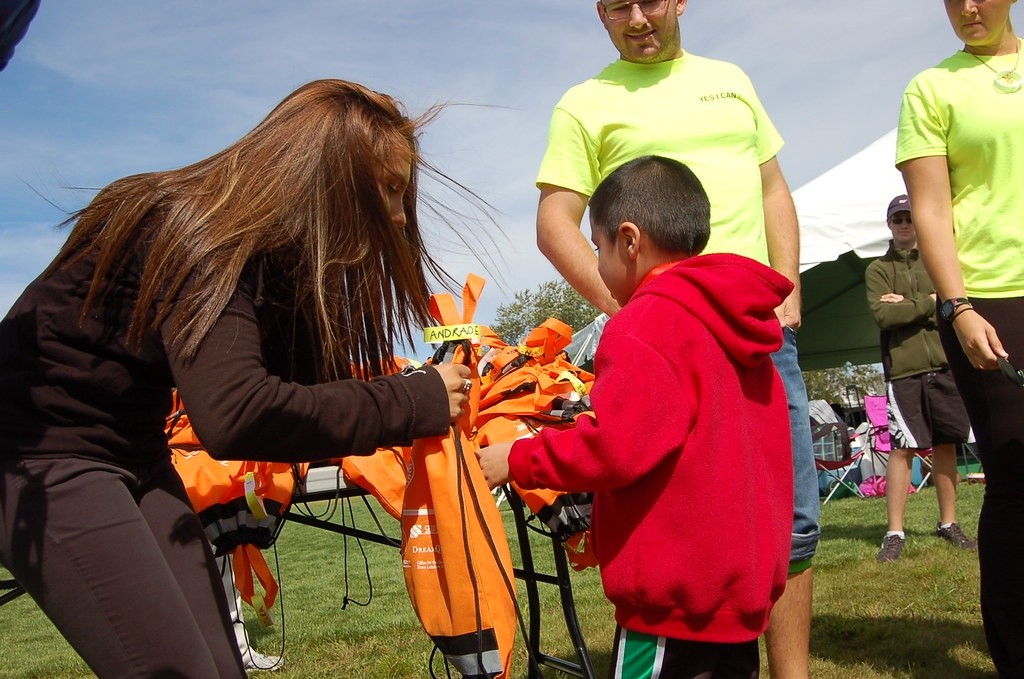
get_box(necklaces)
[966,42,1022,92]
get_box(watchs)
[940,297,972,324]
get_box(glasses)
[890,218,912,225]
[600,0,668,20]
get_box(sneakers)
[877,535,905,562]
[243,652,285,673]
[936,521,975,551]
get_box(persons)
[895,0,1024,679]
[477,156,794,679]
[864,194,974,562]
[0,77,473,679]
[534,0,821,679]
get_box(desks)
[0,452,594,679]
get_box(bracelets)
[950,308,975,324]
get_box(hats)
[887,195,911,218]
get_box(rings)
[463,378,472,393]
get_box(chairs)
[814,424,890,505]
[865,393,935,493]
